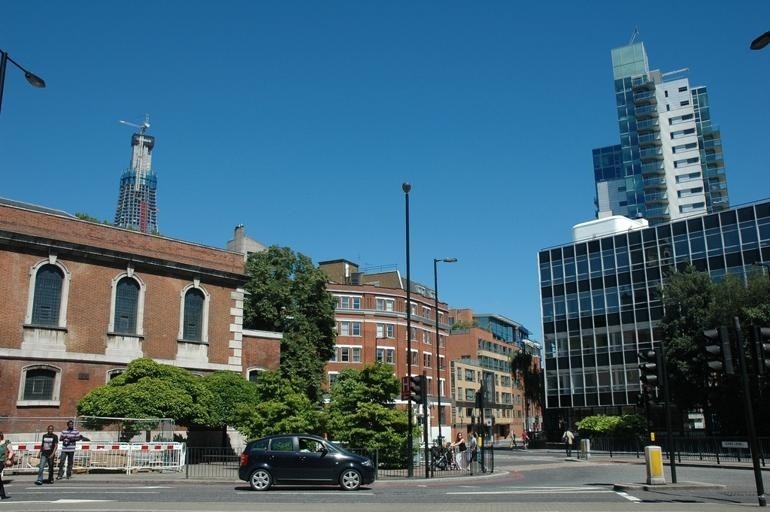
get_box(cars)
[238,434,375,491]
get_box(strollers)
[428,447,462,471]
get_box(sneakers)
[55,476,62,480]
[50,479,53,484]
[67,477,70,480]
[35,480,42,486]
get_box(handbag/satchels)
[564,436,568,443]
[5,449,15,467]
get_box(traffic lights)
[637,394,646,415]
[702,328,724,372]
[409,376,422,404]
[759,327,770,369]
[645,385,656,410]
[637,349,658,384]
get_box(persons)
[0,431,13,499]
[562,429,574,456]
[34,425,58,485]
[55,421,81,480]
[504,427,529,450]
[451,431,482,472]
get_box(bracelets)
[7,458,11,460]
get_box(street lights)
[434,256,458,447]
